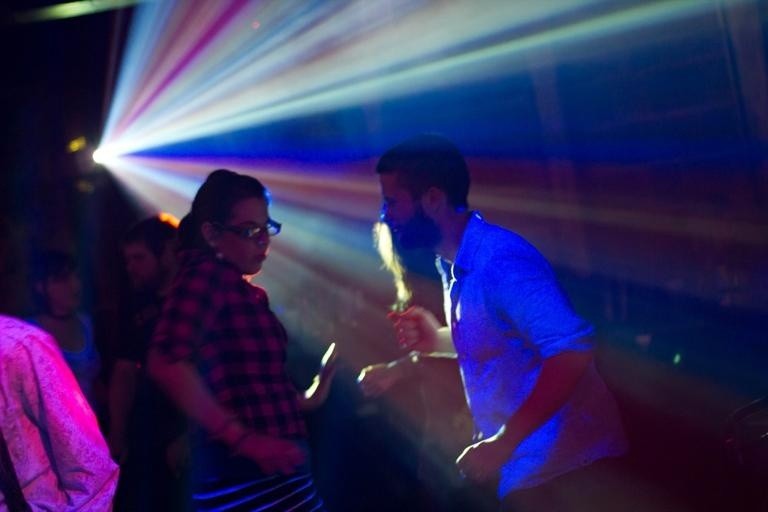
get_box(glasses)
[210,217,281,239]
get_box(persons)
[26,237,113,448]
[0,313,122,512]
[372,131,638,511]
[142,168,343,512]
[355,350,504,512]
[104,211,198,512]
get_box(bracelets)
[229,424,252,458]
[215,409,241,440]
